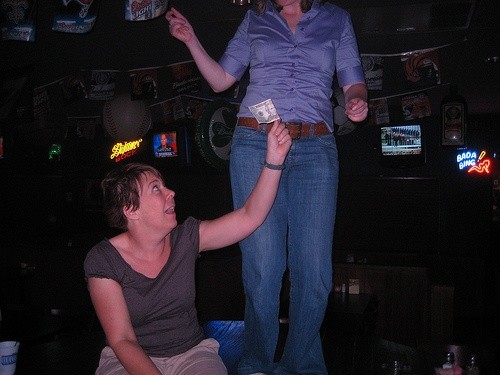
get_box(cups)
[0,341,21,375]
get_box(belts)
[238,117,328,140]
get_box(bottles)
[443,351,479,375]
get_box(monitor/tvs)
[145,125,192,169]
[376,122,428,166]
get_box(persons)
[83,121,293,375]
[165,0,368,375]
[383,130,406,145]
[157,134,174,156]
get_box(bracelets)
[264,161,285,170]
